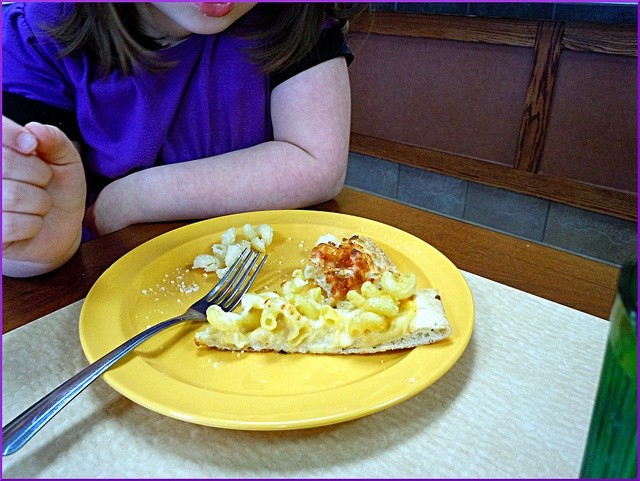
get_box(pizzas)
[193,234,450,355]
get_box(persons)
[1,0,353,280]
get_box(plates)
[77,208,475,432]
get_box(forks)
[1,248,267,460]
